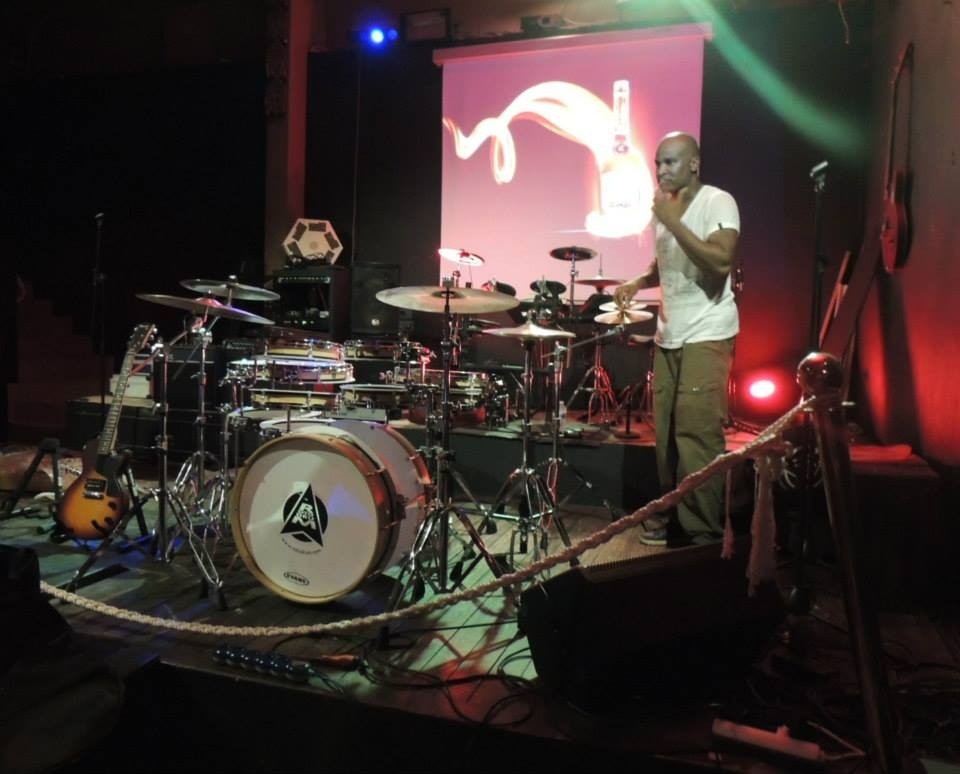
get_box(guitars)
[57,322,158,539]
[879,39,913,273]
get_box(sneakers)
[639,524,688,546]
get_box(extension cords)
[713,720,824,761]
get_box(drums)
[344,340,402,357]
[226,410,323,466]
[265,337,340,360]
[227,359,356,384]
[229,420,432,604]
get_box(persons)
[613,129,740,579]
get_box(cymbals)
[179,279,280,301]
[599,300,648,312]
[481,320,576,341]
[136,294,276,325]
[375,286,520,314]
[550,247,597,261]
[530,280,566,294]
[594,310,654,324]
[438,248,485,266]
[574,276,623,285]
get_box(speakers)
[518,538,748,715]
[0,542,72,656]
